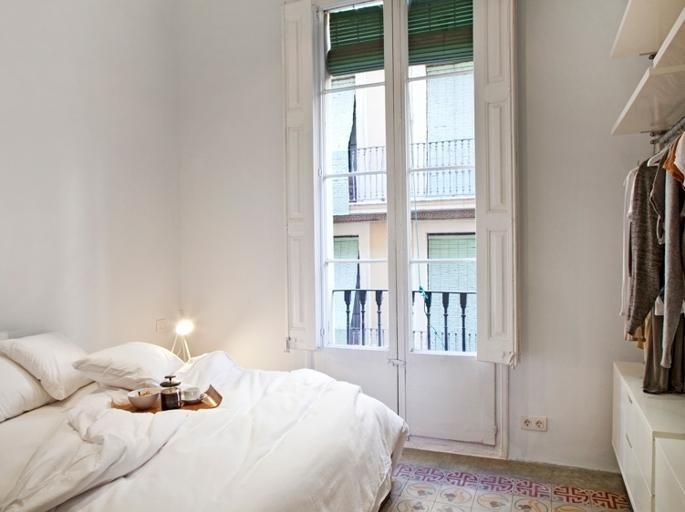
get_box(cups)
[184,387,200,400]
[160,375,181,410]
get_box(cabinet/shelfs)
[604,359,682,512]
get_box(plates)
[181,393,207,403]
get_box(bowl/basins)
[127,388,159,410]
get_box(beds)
[0,331,403,512]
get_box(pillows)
[1,328,182,420]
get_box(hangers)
[614,117,685,193]
